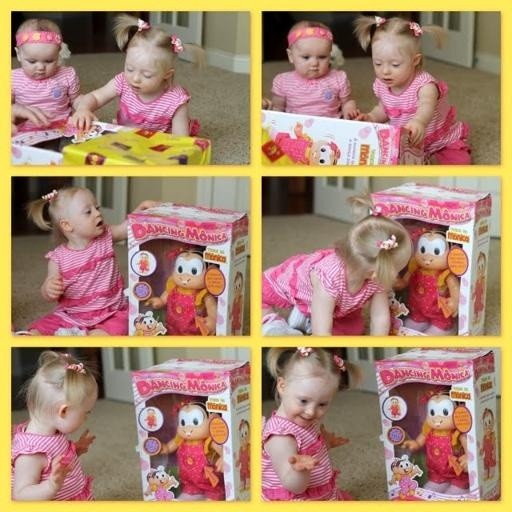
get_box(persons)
[71,13,192,137]
[16,184,161,336]
[259,192,413,338]
[262,98,273,110]
[12,350,98,501]
[263,21,361,120]
[352,15,471,166]
[12,102,55,136]
[12,18,98,131]
[262,347,363,501]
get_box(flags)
[471,251,486,324]
[397,388,469,495]
[155,401,224,501]
[403,228,461,337]
[143,244,218,336]
[236,419,250,491]
[271,122,340,167]
[229,271,244,335]
[479,407,497,480]
